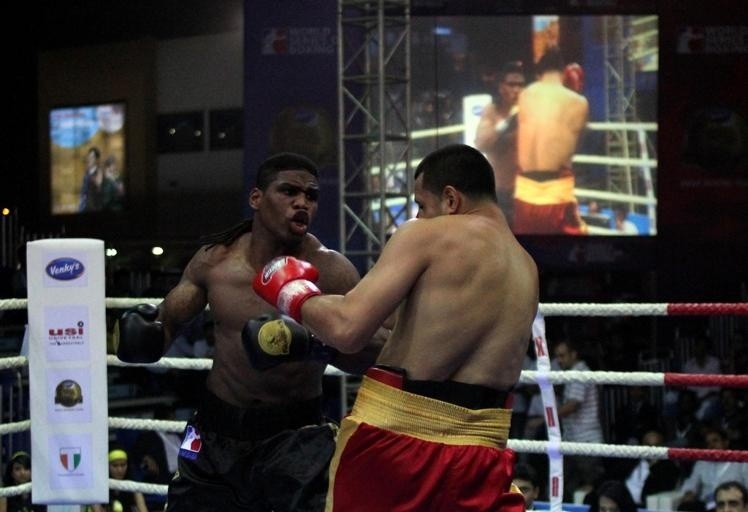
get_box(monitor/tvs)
[49,102,129,216]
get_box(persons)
[1,308,190,512]
[255,147,539,512]
[512,54,589,232]
[582,201,638,235]
[476,69,525,213]
[113,151,388,512]
[509,335,748,512]
[79,148,124,211]
[415,86,463,157]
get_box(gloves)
[252,256,328,324]
[113,303,166,364]
[240,313,310,371]
[565,63,584,92]
